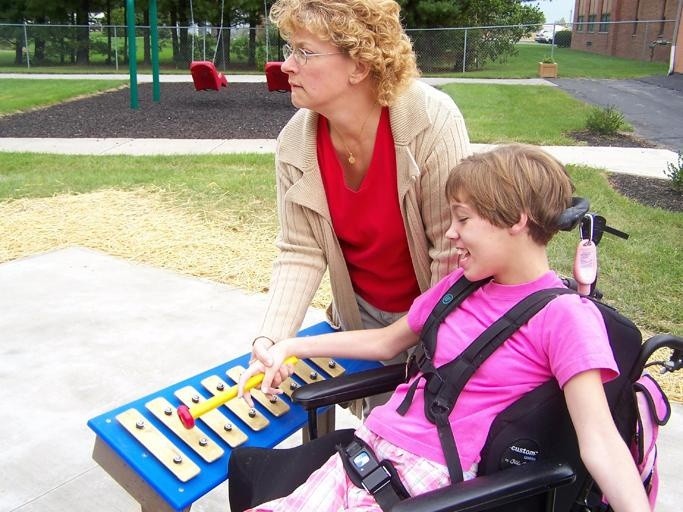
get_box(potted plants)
[538,54,557,79]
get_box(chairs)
[265,61,291,93]
[190,60,228,91]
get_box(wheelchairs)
[228,197,683,512]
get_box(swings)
[190,0,227,92]
[264,1,291,93]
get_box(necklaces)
[336,98,370,172]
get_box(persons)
[246,0,470,430]
[238,143,656,512]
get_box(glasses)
[282,44,347,66]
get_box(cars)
[535,30,552,44]
